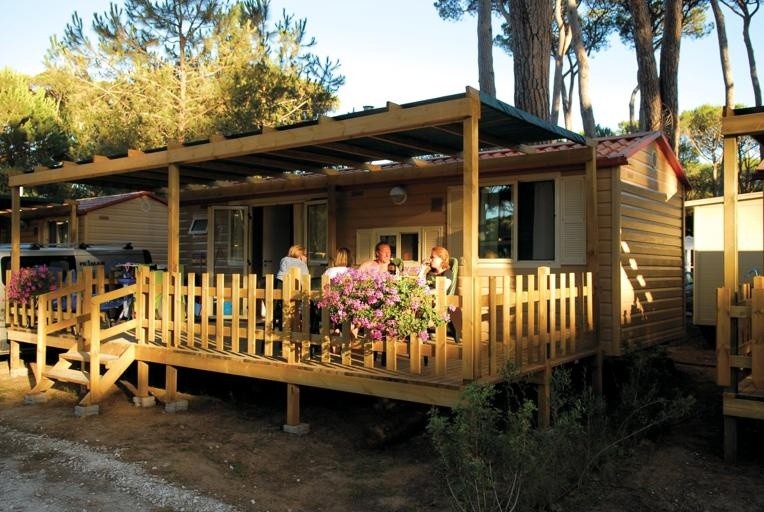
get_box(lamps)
[389,187,407,206]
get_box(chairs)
[446,258,461,343]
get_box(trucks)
[0,242,153,312]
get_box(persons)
[261,244,310,355]
[323,247,352,278]
[417,245,454,307]
[357,241,399,274]
